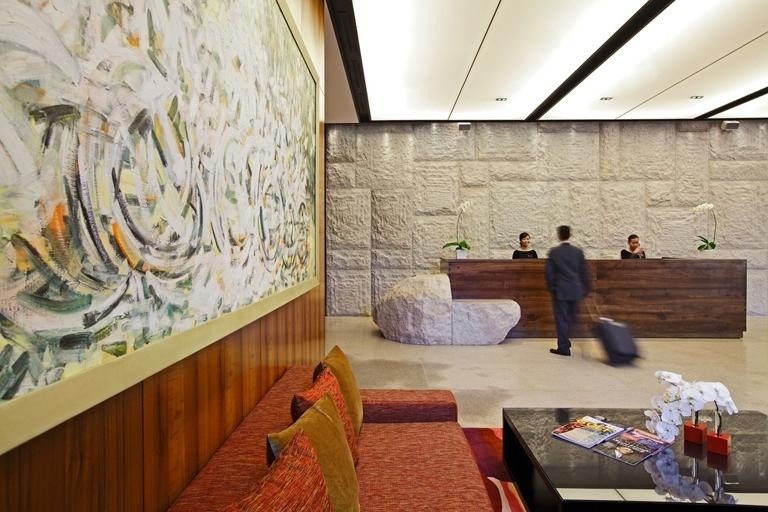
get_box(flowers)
[643,369,699,433]
[441,201,472,250]
[657,381,738,443]
[691,203,716,250]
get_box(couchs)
[169,363,493,511]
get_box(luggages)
[583,295,637,363]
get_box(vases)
[684,419,705,445]
[707,409,733,455]
[456,250,466,259]
[698,250,714,259]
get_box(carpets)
[463,427,528,512]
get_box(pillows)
[313,345,363,441]
[216,427,332,512]
[266,391,359,511]
[289,367,359,467]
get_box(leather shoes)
[550,348,570,356]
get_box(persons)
[603,445,635,459]
[621,233,646,259]
[543,226,593,356]
[512,232,539,259]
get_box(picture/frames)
[1,0,319,457]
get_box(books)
[593,428,675,466]
[551,414,625,449]
[593,413,635,441]
